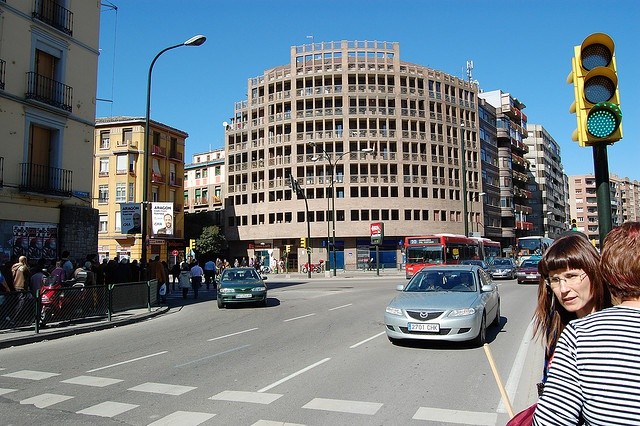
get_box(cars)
[216,267,267,310]
[384,265,500,347]
[490,258,517,280]
[516,256,543,284]
[460,260,489,277]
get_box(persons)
[216,257,222,274]
[172,262,181,290]
[178,266,191,299]
[225,262,231,268]
[31,252,152,323]
[162,261,169,284]
[190,262,203,298]
[241,257,248,267]
[422,274,443,291]
[536,234,606,398]
[203,259,217,289]
[453,273,475,291]
[127,213,141,233]
[254,258,260,271]
[531,221,640,426]
[250,259,255,266]
[7,255,33,326]
[234,258,240,267]
[219,259,227,269]
[0,270,11,293]
[4,254,18,270]
[149,256,168,304]
[157,214,173,234]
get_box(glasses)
[545,272,586,285]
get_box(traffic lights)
[190,239,195,250]
[571,217,577,231]
[286,245,290,253]
[567,32,623,148]
[301,238,306,249]
[307,246,312,255]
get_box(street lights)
[307,141,373,276]
[139,35,206,281]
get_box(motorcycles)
[303,260,324,273]
[38,268,94,329]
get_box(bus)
[515,236,555,267]
[404,233,502,279]
